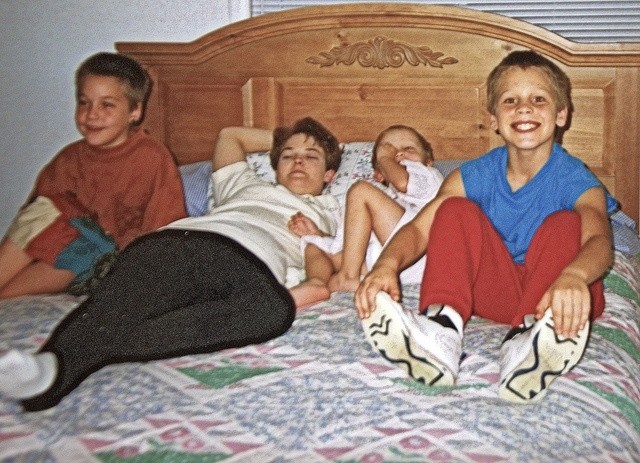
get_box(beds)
[0,2,639,462]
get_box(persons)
[1,52,188,301]
[351,50,622,405]
[286,125,446,309]
[1,116,342,413]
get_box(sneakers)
[361,291,464,387]
[498,306,590,404]
